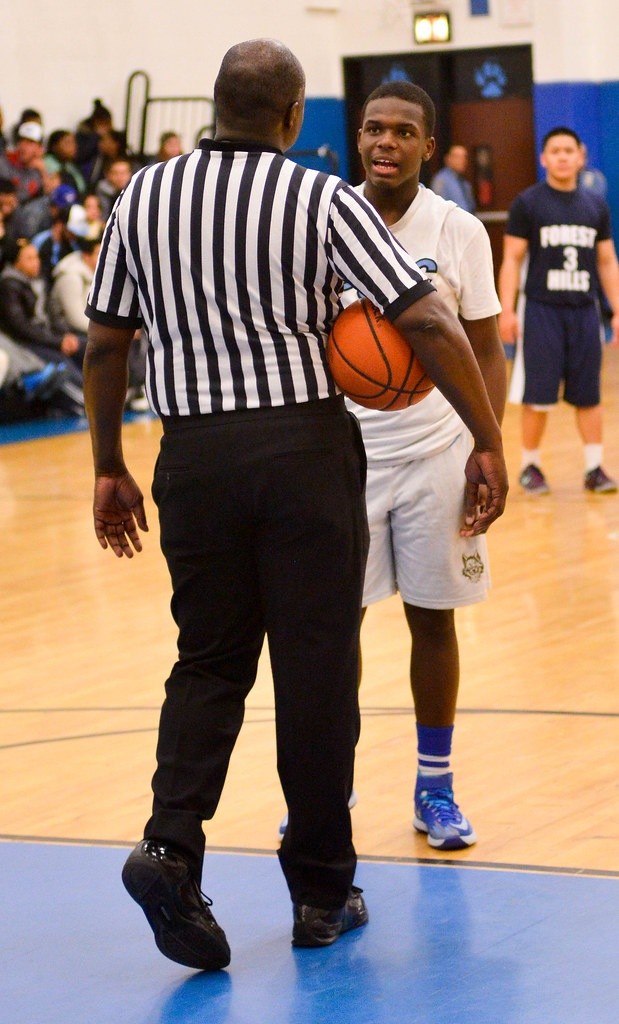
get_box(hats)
[19,121,44,141]
[51,184,74,208]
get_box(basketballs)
[326,297,438,415]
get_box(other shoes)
[518,464,546,492]
[586,467,614,494]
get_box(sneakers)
[291,890,367,946]
[276,792,355,841]
[413,787,475,849]
[121,839,231,970]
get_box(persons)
[278,80,506,849]
[80,39,510,973]
[0,98,185,417]
[430,126,619,494]
